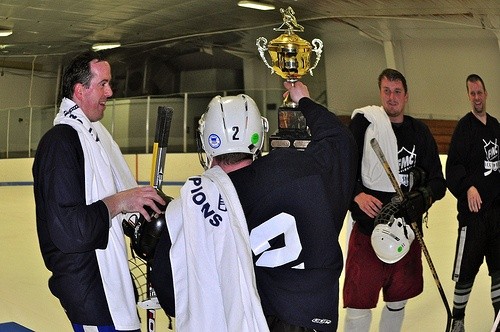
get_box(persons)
[32,50,166,332]
[152,81,359,332]
[343,68,446,332]
[445,74,500,332]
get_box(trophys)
[256,7,324,150]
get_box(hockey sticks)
[369,138,453,332]
[141,106,174,332]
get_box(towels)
[52,96,143,320]
[163,164,270,332]
[350,103,401,193]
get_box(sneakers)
[451,318,466,332]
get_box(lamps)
[92,42,120,51]
[237,0,276,10]
[0,29,12,37]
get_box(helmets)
[193,93,271,172]
[120,196,175,261]
[370,201,417,265]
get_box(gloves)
[398,185,430,223]
